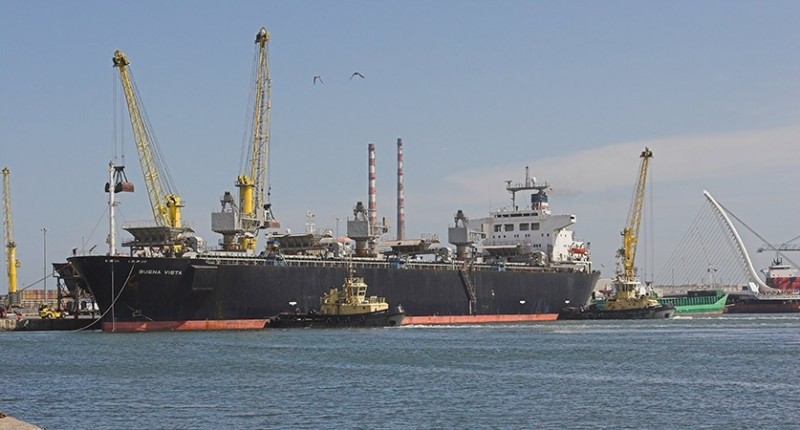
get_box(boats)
[0,166,25,319]
[262,245,407,330]
[555,142,678,321]
[656,291,732,318]
[50,137,602,333]
[725,245,800,315]
[570,241,586,255]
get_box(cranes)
[208,24,283,260]
[110,49,206,258]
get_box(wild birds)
[313,75,323,85]
[349,72,364,80]
